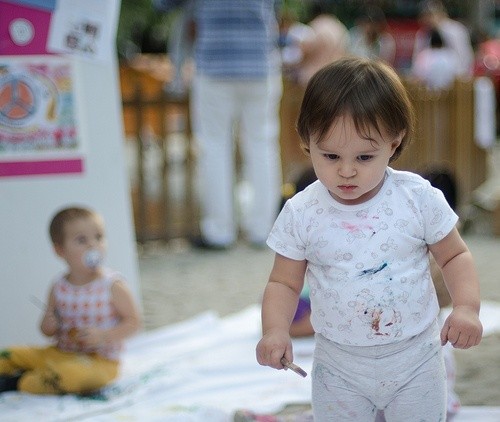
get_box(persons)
[160,0,283,248]
[0,207,140,391]
[271,0,500,88]
[255,58,483,421]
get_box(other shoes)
[189,233,224,251]
